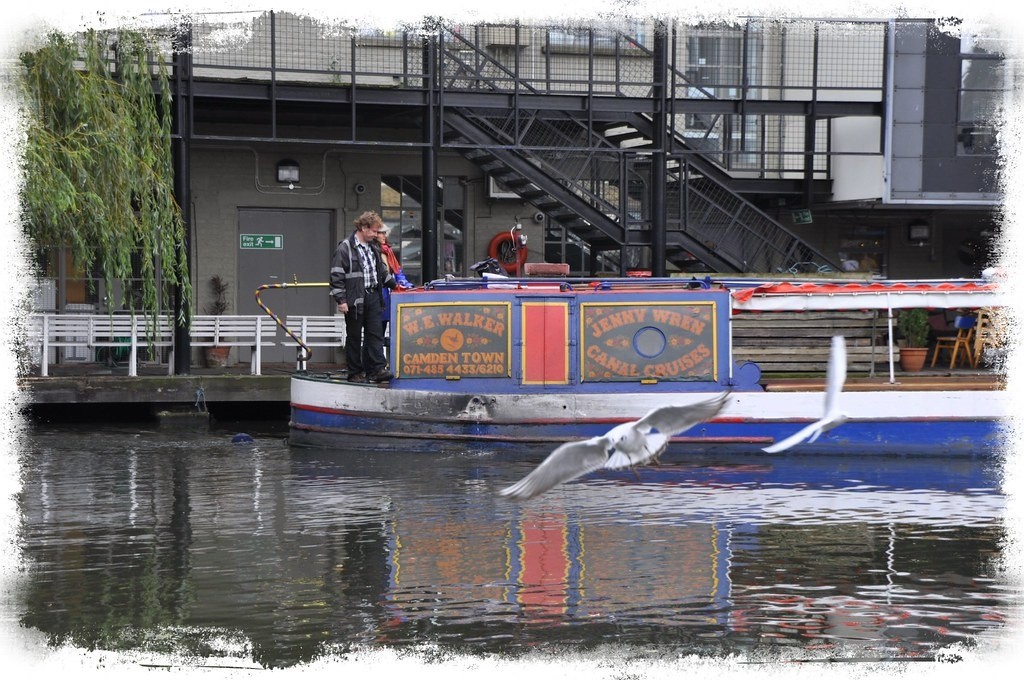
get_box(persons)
[329,211,406,382]
[362,224,414,374]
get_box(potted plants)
[899,307,931,373]
[202,275,233,368]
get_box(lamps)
[909,218,931,242]
[277,157,300,183]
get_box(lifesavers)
[488,231,528,273]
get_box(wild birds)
[761,333,853,455]
[493,388,733,503]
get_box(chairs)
[930,309,1008,370]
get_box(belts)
[364,288,376,294]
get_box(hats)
[377,224,389,232]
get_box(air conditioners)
[484,156,542,200]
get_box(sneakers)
[347,374,369,383]
[371,367,394,381]
